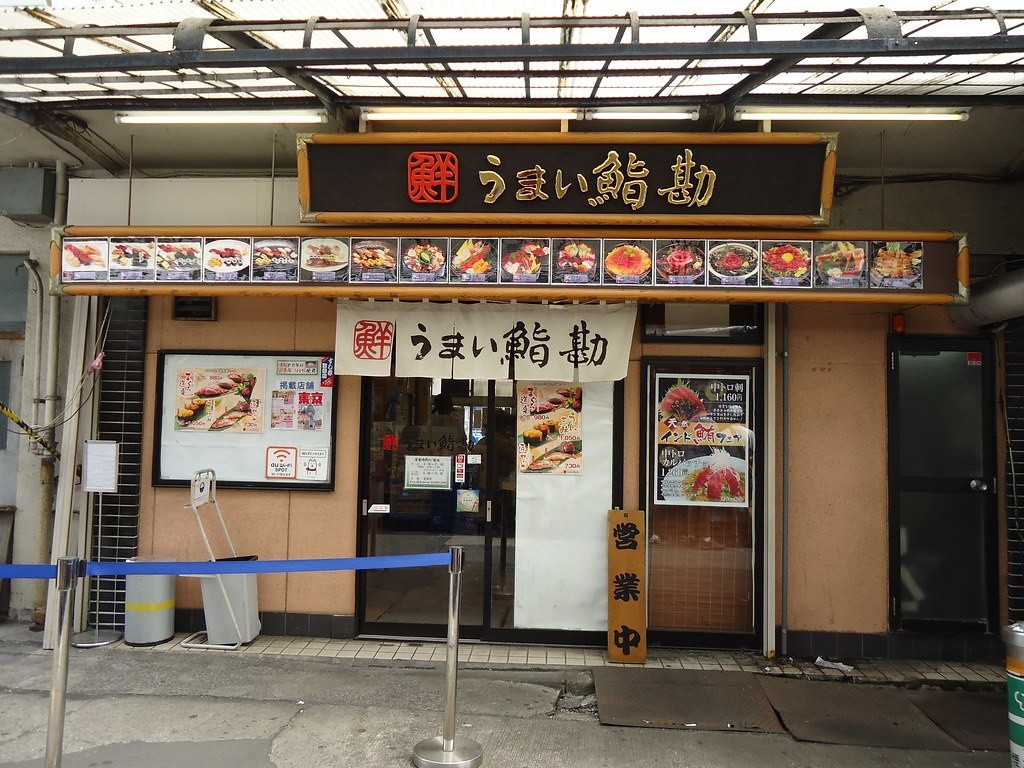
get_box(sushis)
[178,382,231,422]
[157,244,299,268]
[523,420,558,446]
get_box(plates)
[158,242,201,271]
[301,239,348,271]
[205,240,250,273]
[254,240,298,269]
[110,243,155,270]
[662,456,745,500]
[352,241,397,273]
[64,241,107,272]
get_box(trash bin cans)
[123,556,176,647]
[201,556,261,644]
[1000,621,1024,768]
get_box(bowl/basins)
[500,250,540,281]
[403,244,446,274]
[604,243,652,282]
[556,241,598,282]
[656,243,705,282]
[709,243,759,279]
[818,264,863,287]
[450,240,497,280]
[761,242,811,283]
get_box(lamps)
[734,105,974,121]
[115,108,325,125]
[358,104,702,123]
